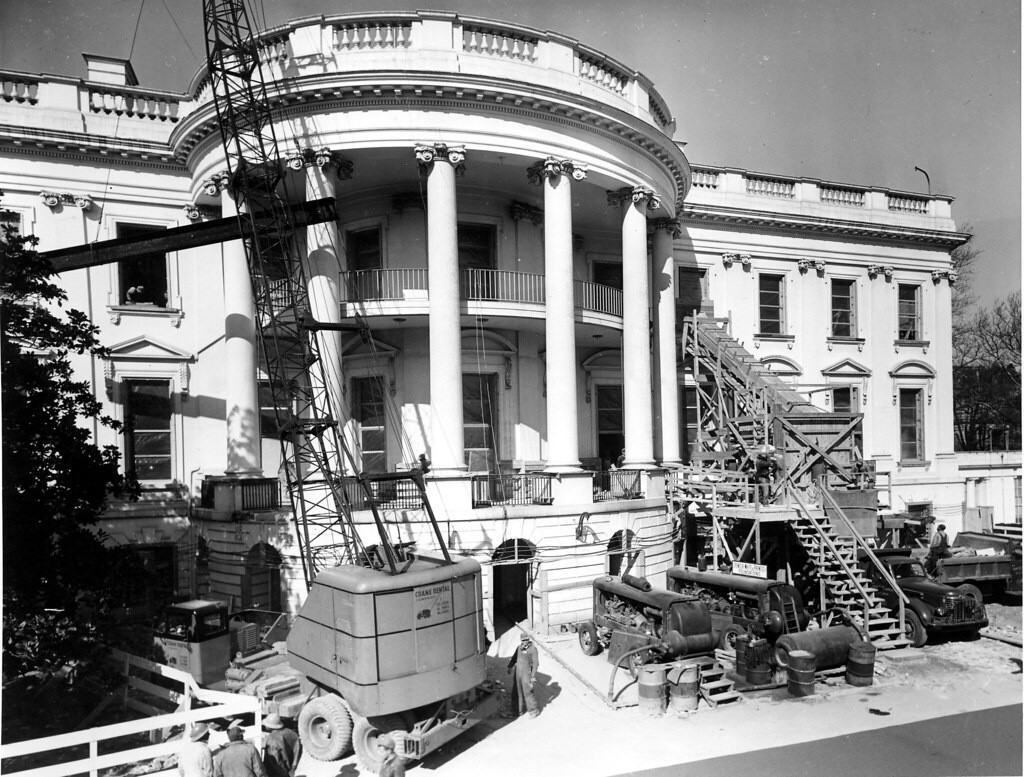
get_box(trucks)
[841,546,990,649]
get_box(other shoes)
[530,710,540,718]
[514,712,524,716]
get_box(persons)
[756,450,775,506]
[850,458,874,483]
[506,633,541,717]
[179,711,303,777]
[927,523,949,570]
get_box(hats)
[228,727,245,741]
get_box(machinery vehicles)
[199,0,510,777]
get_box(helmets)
[190,723,209,742]
[520,633,530,641]
[261,712,283,729]
